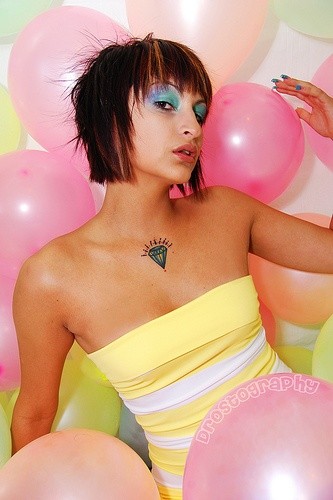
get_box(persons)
[9,31,333,499]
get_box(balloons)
[0,0,333,499]
